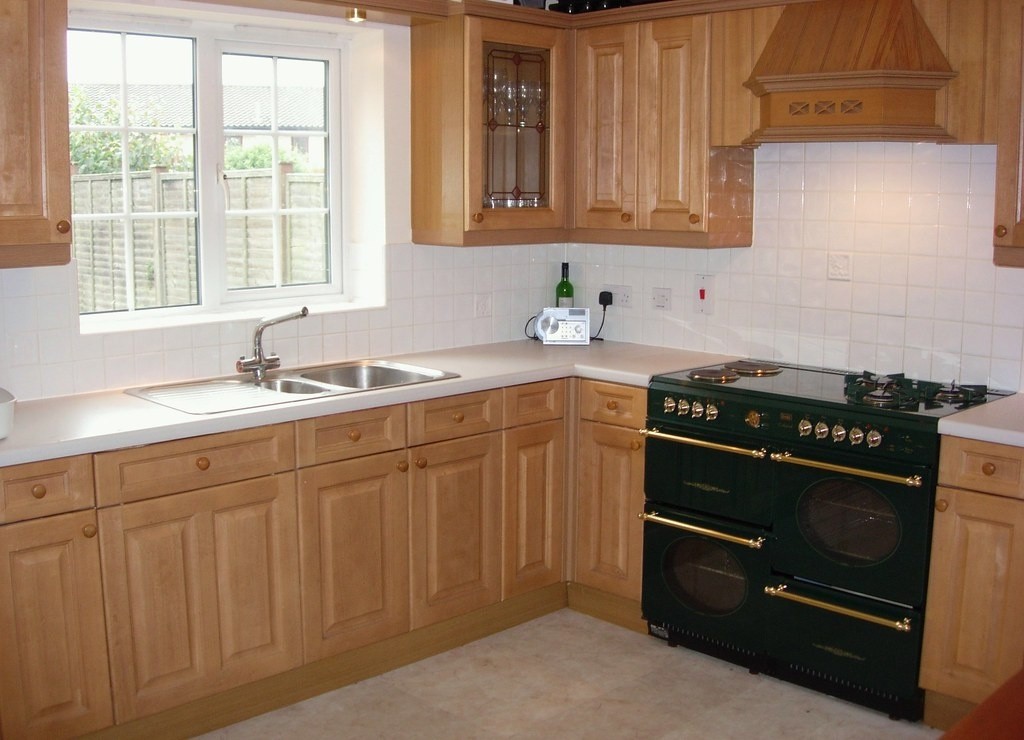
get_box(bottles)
[556,262,574,308]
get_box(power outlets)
[596,285,633,307]
[651,288,671,309]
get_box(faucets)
[236,305,309,382]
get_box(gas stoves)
[646,358,1018,471]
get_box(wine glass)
[483,65,550,129]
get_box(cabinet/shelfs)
[411,0,1024,269]
[916,433,1024,731]
[0,0,73,268]
[0,374,646,740]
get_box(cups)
[482,196,546,209]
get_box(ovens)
[638,420,938,726]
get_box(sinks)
[254,378,331,395]
[296,359,460,394]
